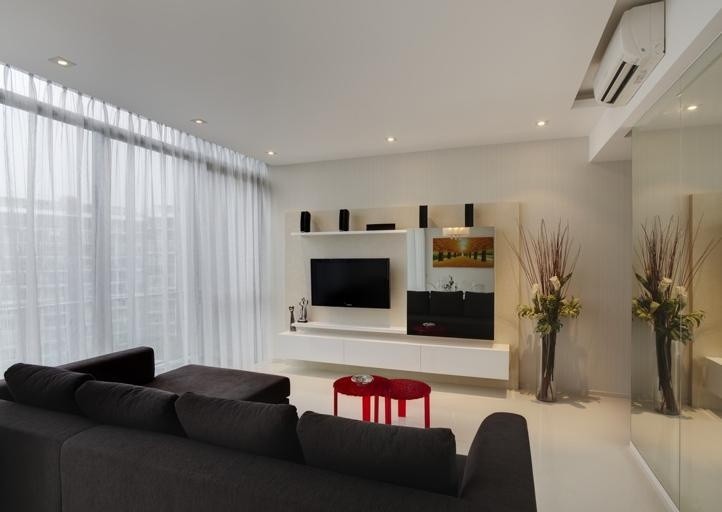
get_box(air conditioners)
[592,1,666,109]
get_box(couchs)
[407,298,493,340]
[1,346,538,512]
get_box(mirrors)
[627,91,722,511]
[405,226,496,341]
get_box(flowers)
[500,215,584,402]
[629,212,722,413]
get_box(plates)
[351,374,375,385]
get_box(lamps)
[442,227,470,241]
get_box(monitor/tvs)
[310,257,391,309]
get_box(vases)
[656,336,681,415]
[534,333,558,402]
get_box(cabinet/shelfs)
[279,329,510,385]
